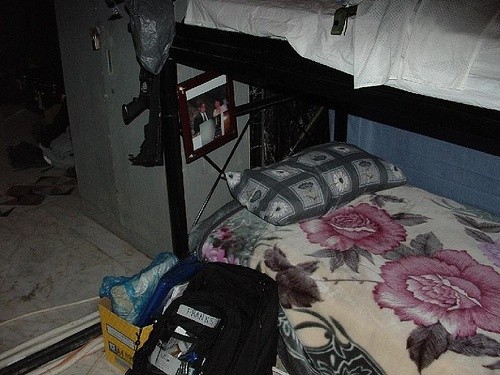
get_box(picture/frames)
[178,72,239,165]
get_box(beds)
[188,180,500,375]
[166,0,500,158]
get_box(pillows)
[224,141,407,227]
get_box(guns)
[113,1,164,168]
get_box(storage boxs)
[96,295,161,375]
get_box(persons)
[212,99,228,117]
[193,102,212,134]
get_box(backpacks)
[124,261,280,375]
